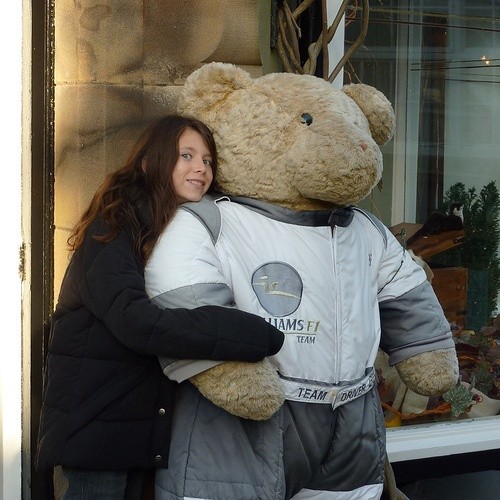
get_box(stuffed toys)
[141,61,461,500]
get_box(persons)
[38,116,286,500]
